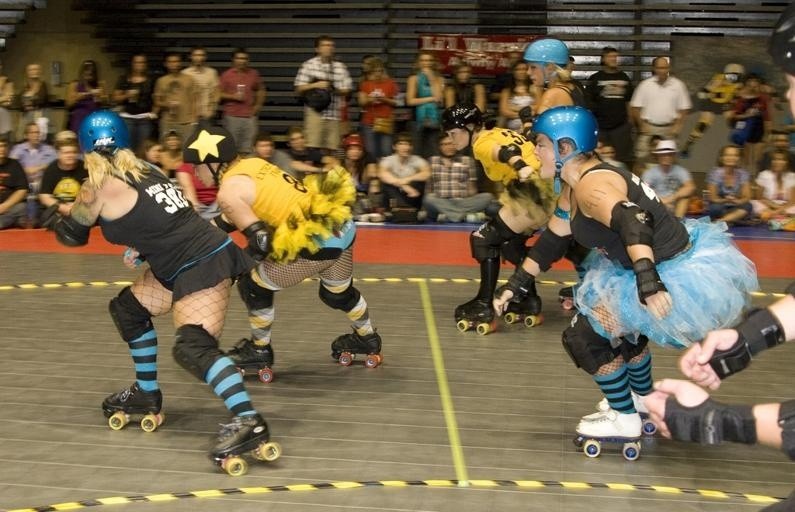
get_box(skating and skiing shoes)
[574,410,642,460]
[101,383,166,433]
[556,282,588,312]
[503,295,545,328]
[330,332,383,369]
[208,414,281,478]
[452,302,498,336]
[597,392,657,436]
[226,339,274,383]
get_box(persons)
[1,37,794,231]
[184,121,382,384]
[492,105,757,462]
[645,7,794,512]
[440,102,590,335]
[39,110,279,477]
[504,38,588,310]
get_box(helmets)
[523,39,569,67]
[441,102,482,129]
[342,132,367,147]
[530,106,599,166]
[79,111,129,154]
[183,125,236,165]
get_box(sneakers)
[358,209,490,226]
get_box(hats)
[723,63,748,84]
[305,84,332,114]
[52,178,82,203]
[652,139,679,156]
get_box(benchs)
[0,1,795,184]
